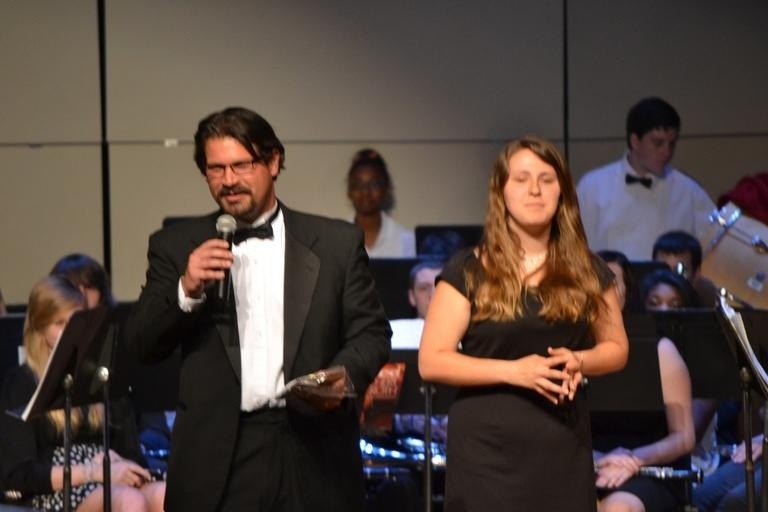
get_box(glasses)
[352,184,383,189]
[205,157,265,178]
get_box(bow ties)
[624,172,653,189]
[230,221,273,247]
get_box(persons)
[406,261,444,320]
[640,270,699,311]
[574,249,699,511]
[574,96,716,257]
[346,148,417,257]
[1,277,167,512]
[47,254,173,481]
[649,232,703,282]
[415,135,626,511]
[119,106,398,511]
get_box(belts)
[238,408,291,425]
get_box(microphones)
[209,214,237,308]
